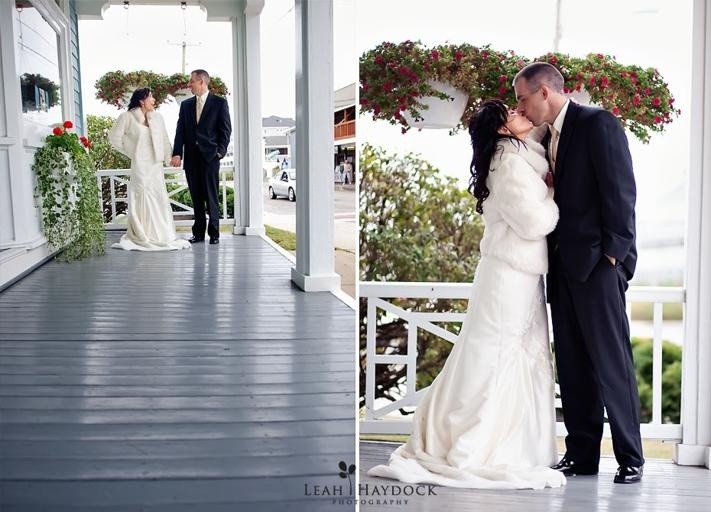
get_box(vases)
[48,151,77,175]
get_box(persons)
[109,86,193,253]
[513,62,647,485]
[281,158,288,169]
[364,97,567,492]
[341,159,352,185]
[171,68,232,244]
[272,158,280,175]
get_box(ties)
[550,124,560,162]
[196,96,203,124]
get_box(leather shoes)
[188,235,205,243]
[550,456,599,476]
[614,463,643,484]
[210,235,219,244]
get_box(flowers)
[29,120,107,262]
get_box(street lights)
[182,41,186,75]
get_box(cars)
[268,168,297,201]
